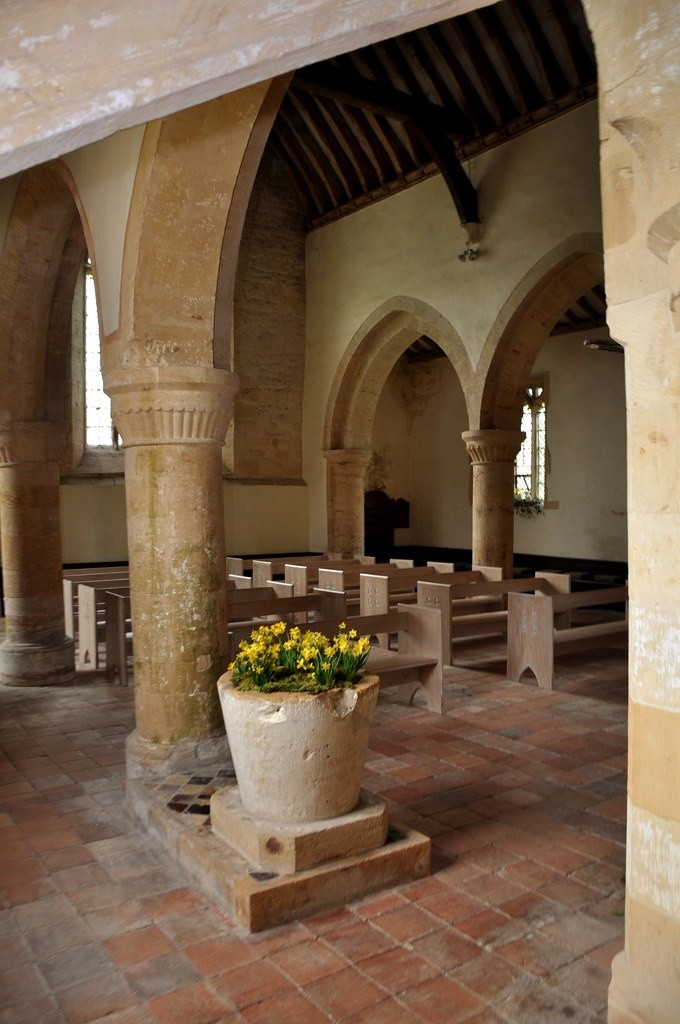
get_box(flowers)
[222,619,373,697]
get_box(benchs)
[64,565,134,688]
[226,552,629,715]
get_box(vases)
[215,667,380,820]
